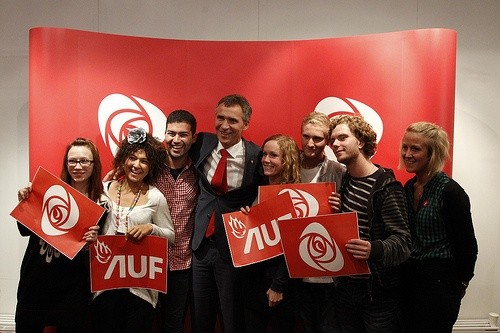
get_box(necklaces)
[114,181,145,235]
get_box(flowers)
[127,126,147,143]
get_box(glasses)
[66,158,93,167]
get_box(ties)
[205,149,229,238]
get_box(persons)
[287,112,348,333]
[90,132,177,333]
[326,114,411,333]
[240,134,303,333]
[15,136,110,333]
[398,122,479,333]
[144,110,200,333]
[102,93,270,333]
